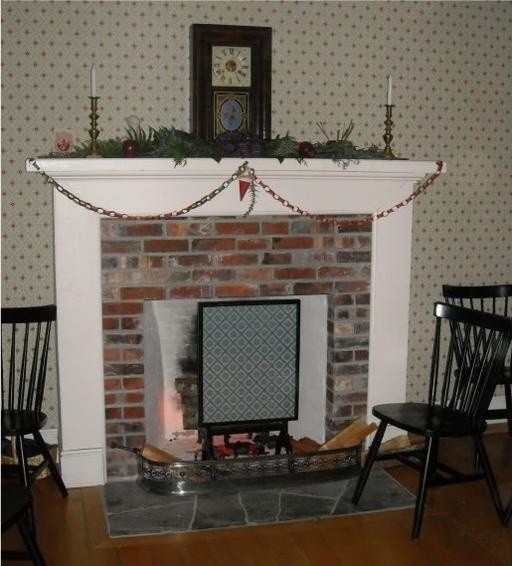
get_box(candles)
[387,72,392,104]
[90,63,96,97]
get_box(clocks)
[191,23,271,140]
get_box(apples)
[123,139,138,157]
[299,141,313,158]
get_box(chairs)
[352,301,512,541]
[1,304,69,566]
[442,284,512,468]
